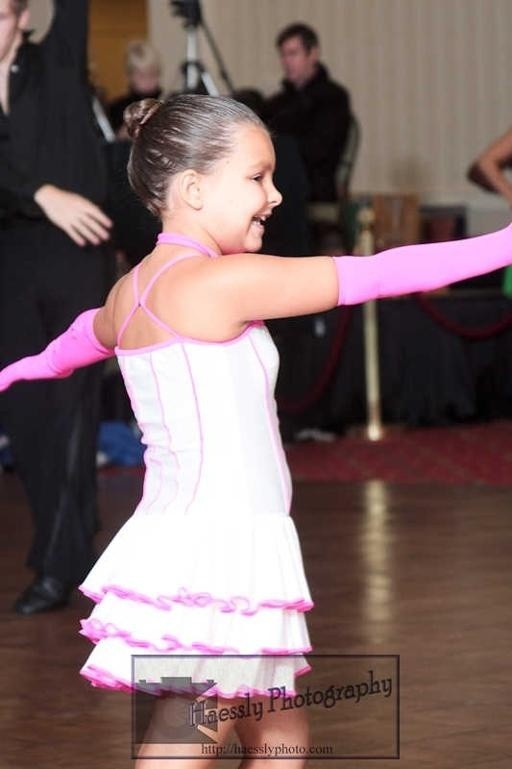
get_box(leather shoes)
[13,557,92,615]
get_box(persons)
[264,22,351,446]
[467,126,512,300]
[0,93,512,769]
[1,1,118,620]
[106,37,210,265]
